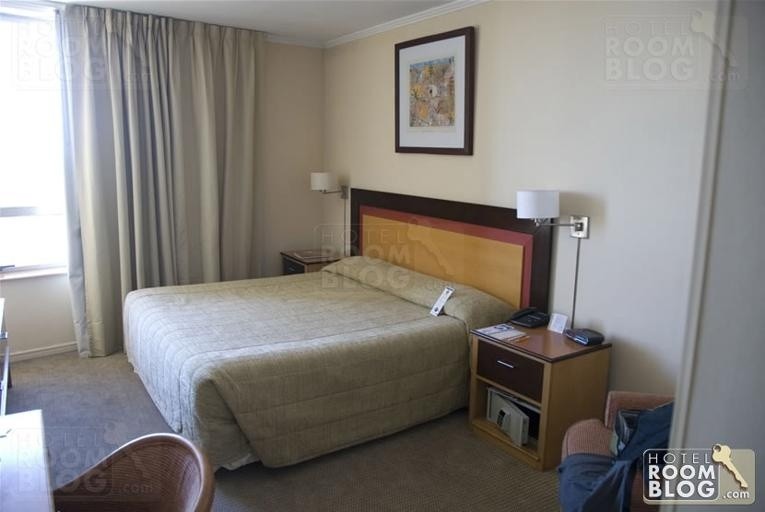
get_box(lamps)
[517,189,589,238]
[310,172,347,198]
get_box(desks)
[0,408,60,512]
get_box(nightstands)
[469,321,611,471]
[280,249,342,275]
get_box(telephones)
[508,307,550,328]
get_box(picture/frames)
[395,27,474,155]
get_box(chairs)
[561,390,675,511]
[53,432,215,512]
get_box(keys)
[711,442,748,490]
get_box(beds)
[123,189,553,475]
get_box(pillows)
[319,256,517,335]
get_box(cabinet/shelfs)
[0,298,13,415]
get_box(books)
[294,251,327,259]
